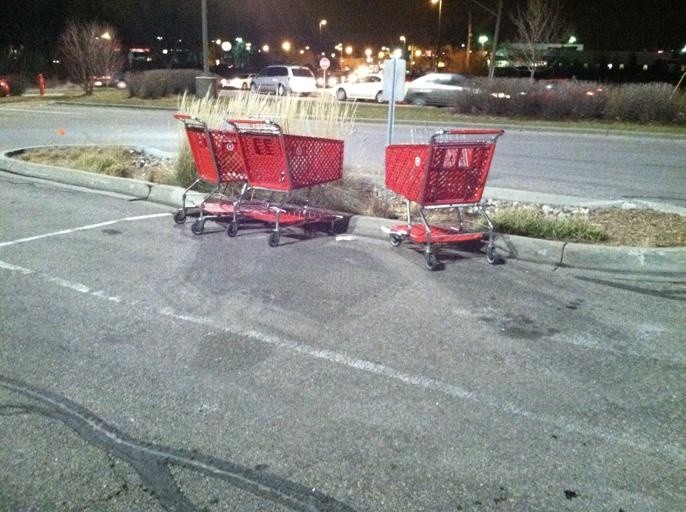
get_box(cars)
[331,73,603,107]
[223,64,316,96]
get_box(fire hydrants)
[36,74,46,95]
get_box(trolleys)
[384,129,504,270]
[173,114,345,247]
